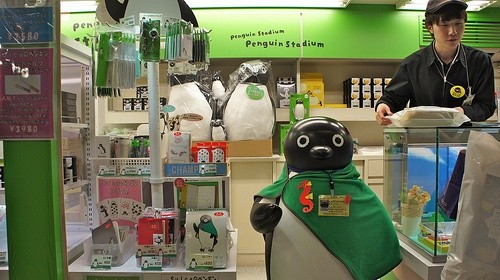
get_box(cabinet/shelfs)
[0,32,500,280]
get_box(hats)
[425,0,468,17]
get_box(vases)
[401,205,423,238]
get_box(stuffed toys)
[166,57,278,141]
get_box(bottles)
[114,136,150,158]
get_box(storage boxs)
[228,138,273,157]
[82,230,138,267]
[168,130,192,165]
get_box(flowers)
[397,182,432,206]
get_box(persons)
[374,0,497,122]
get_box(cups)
[401,215,422,238]
